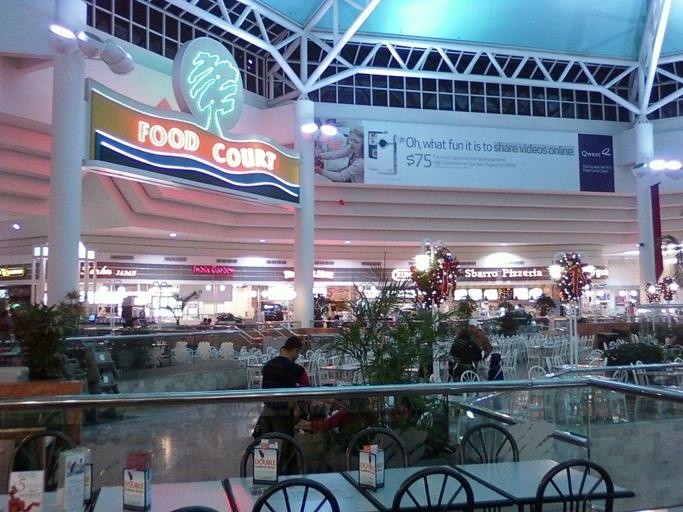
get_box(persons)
[488,353,504,380]
[314,125,364,185]
[251,336,313,476]
[515,303,525,317]
[625,302,635,316]
[200,318,211,326]
[448,325,492,382]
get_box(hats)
[469,319,478,327]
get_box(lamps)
[47,22,135,76]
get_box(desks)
[91,457,637,510]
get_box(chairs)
[429,334,683,418]
[7,426,79,493]
[247,345,378,385]
[241,419,616,510]
